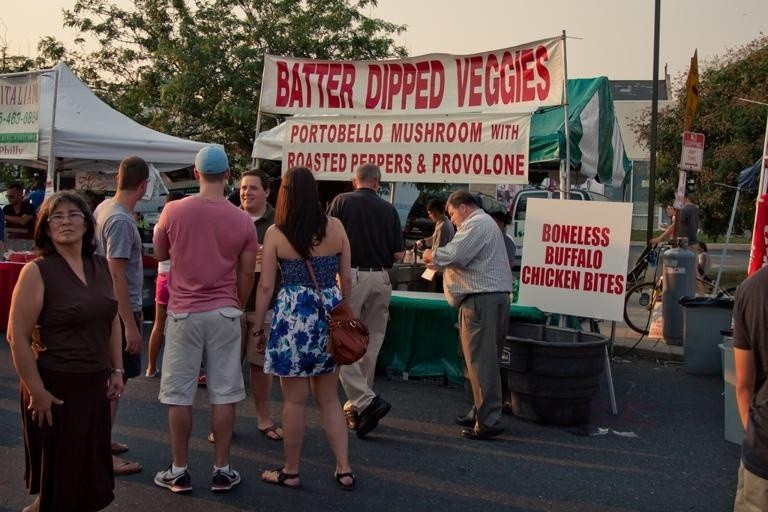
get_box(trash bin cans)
[678,297,734,374]
[719,330,747,446]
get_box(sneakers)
[207,463,243,494]
[355,393,392,439]
[197,375,208,386]
[144,366,161,379]
[343,407,361,431]
[151,460,194,495]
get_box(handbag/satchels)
[322,293,372,368]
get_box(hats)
[193,142,231,176]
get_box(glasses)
[42,211,89,224]
[4,194,16,200]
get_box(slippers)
[110,439,130,454]
[111,453,144,476]
[255,419,283,442]
[206,425,238,444]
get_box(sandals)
[256,465,304,489]
[333,466,359,491]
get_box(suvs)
[401,191,512,251]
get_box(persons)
[7,192,125,512]
[649,187,705,298]
[733,265,768,512]
[698,241,711,279]
[208,167,356,488]
[327,163,405,437]
[415,190,513,437]
[1,177,46,239]
[146,146,258,491]
[91,155,149,473]
[659,203,675,243]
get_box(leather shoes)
[454,413,475,427]
[460,424,507,442]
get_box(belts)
[350,263,393,272]
[458,291,512,309]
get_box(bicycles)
[622,238,737,335]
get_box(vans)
[506,189,614,269]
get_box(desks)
[0,247,161,334]
[375,290,583,384]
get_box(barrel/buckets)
[718,335,745,446]
